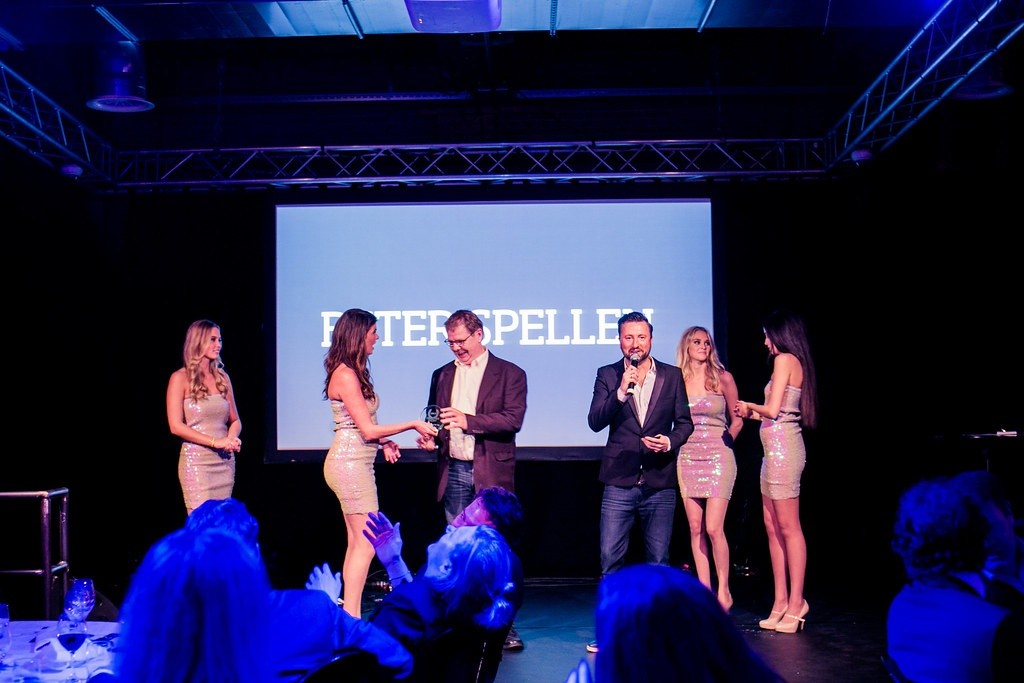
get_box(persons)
[112,498,414,683]
[591,564,786,683]
[323,309,438,619]
[733,308,816,633]
[416,309,528,650]
[306,485,523,645]
[886,470,1024,683]
[586,312,695,652]
[677,326,744,614]
[166,320,242,516]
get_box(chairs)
[301,589,524,683]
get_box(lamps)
[86,39,155,115]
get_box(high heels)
[775,599,809,633]
[759,604,789,630]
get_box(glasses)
[443,331,476,346]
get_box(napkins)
[34,624,61,653]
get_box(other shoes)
[586,640,598,653]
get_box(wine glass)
[0,578,116,683]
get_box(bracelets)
[211,437,214,447]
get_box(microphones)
[627,353,641,399]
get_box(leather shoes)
[503,626,523,649]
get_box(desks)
[0,621,118,683]
[935,434,1024,474]
